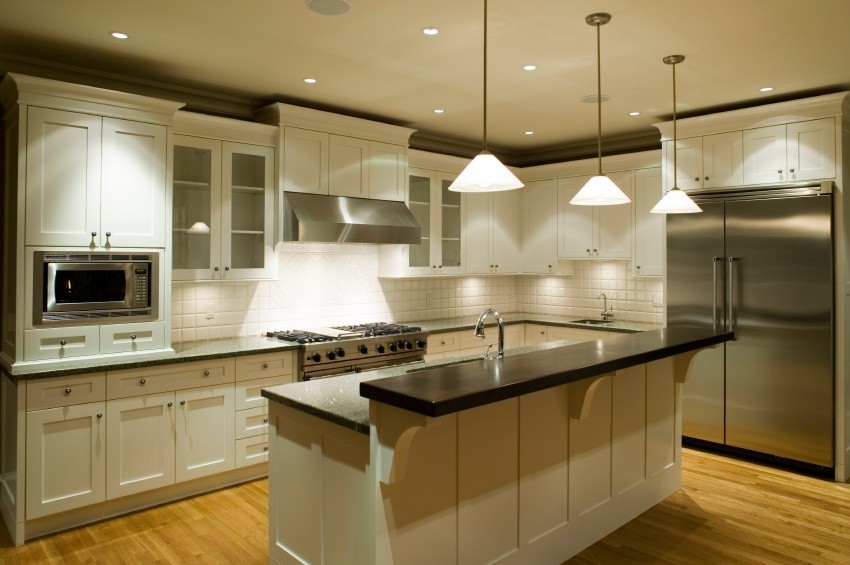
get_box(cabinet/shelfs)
[467,189,520,275]
[236,350,293,469]
[667,131,743,191]
[557,169,633,259]
[633,167,664,277]
[107,356,236,500]
[26,372,107,520]
[520,180,575,276]
[26,105,165,247]
[171,133,275,282]
[424,325,608,360]
[283,126,405,202]
[378,167,466,278]
[742,116,837,186]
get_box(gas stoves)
[266,322,429,366]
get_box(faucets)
[597,294,614,320]
[474,307,506,361]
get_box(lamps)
[570,14,633,206]
[448,0,524,192]
[188,151,210,234]
[649,55,702,213]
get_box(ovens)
[301,350,427,381]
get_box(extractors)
[282,191,422,245]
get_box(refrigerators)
[666,181,834,477]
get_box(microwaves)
[25,246,164,329]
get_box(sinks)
[568,319,614,324]
